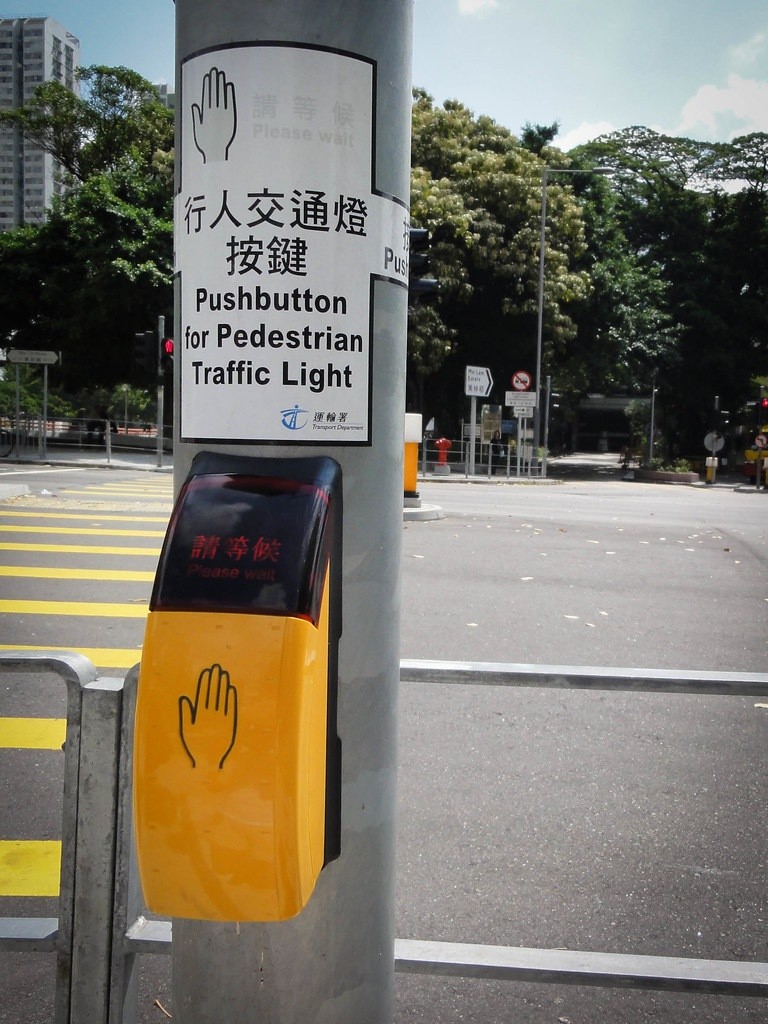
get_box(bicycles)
[0,428,15,457]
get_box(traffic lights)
[760,398,768,409]
[158,336,174,379]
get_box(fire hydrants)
[434,438,453,465]
